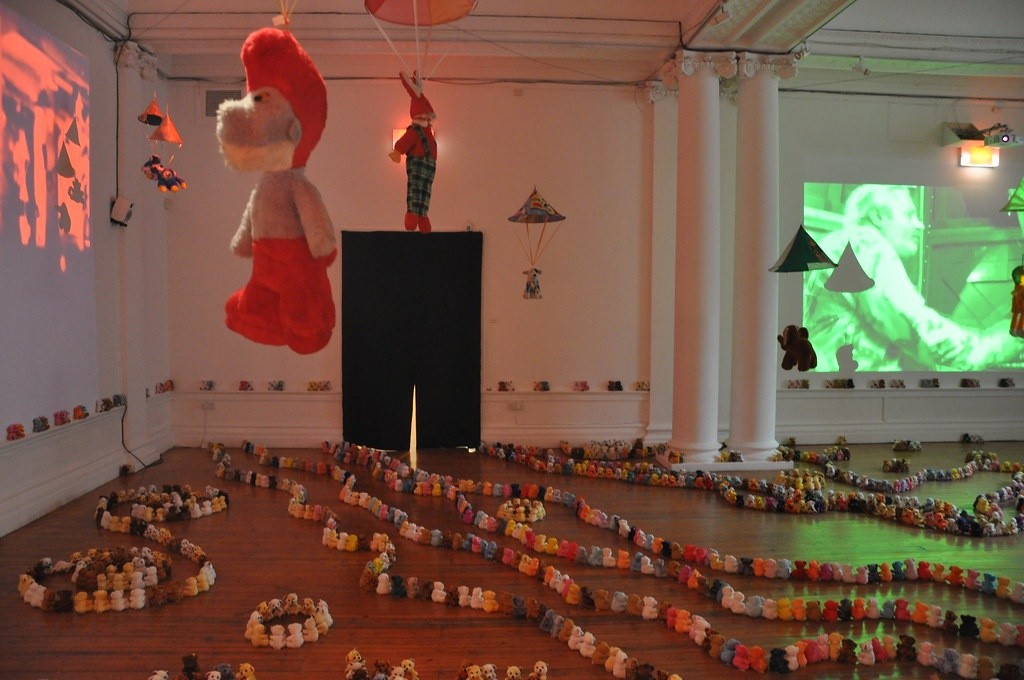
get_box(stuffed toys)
[520,267,544,300]
[1007,265,1024,338]
[214,25,338,356]
[776,323,819,372]
[0,375,1024,680]
[385,69,437,234]
[139,154,192,193]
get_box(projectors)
[984,132,1024,147]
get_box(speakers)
[111,197,136,224]
